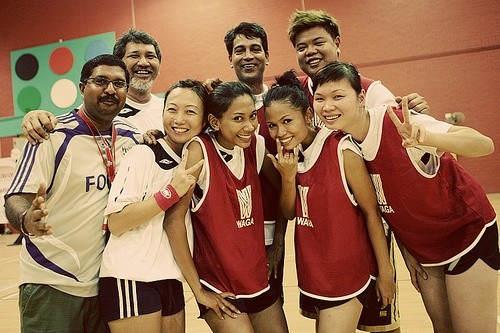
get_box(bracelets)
[154,184,180,211]
[19,209,37,239]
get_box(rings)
[267,263,271,267]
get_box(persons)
[164,82,288,333]
[4,54,145,333]
[21,29,167,146]
[204,10,430,333]
[98,78,210,333]
[140,21,287,305]
[262,68,396,333]
[313,61,500,333]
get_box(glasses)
[83,77,130,89]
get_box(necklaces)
[77,109,117,183]
